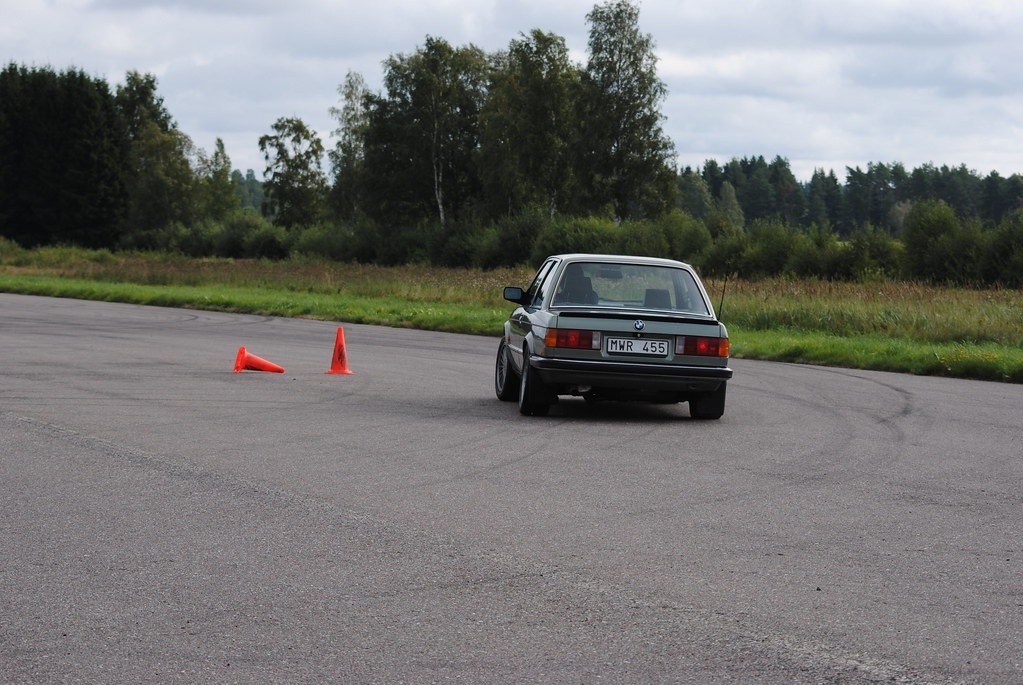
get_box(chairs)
[562,275,593,303]
[643,289,672,309]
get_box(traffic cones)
[234,347,286,373]
[325,326,353,375]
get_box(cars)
[491,252,733,422]
